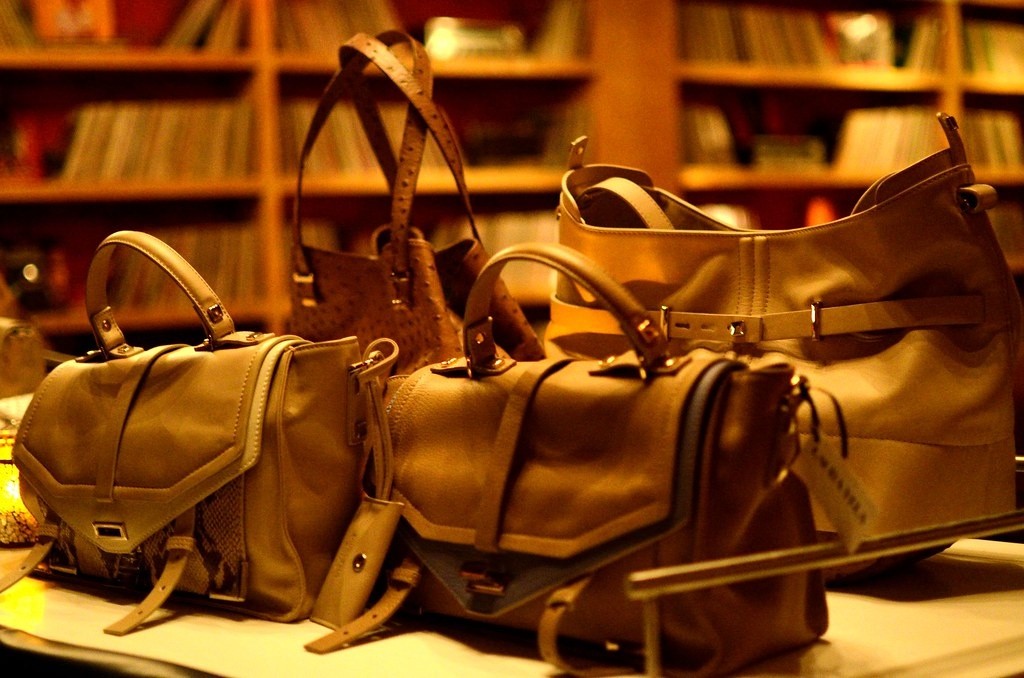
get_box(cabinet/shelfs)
[638,0,1023,546]
[0,0,636,372]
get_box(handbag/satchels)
[277,30,553,369]
[8,231,365,621]
[551,131,1024,575]
[384,242,828,663]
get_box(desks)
[3,392,1024,677]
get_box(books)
[284,205,560,304]
[46,100,251,183]
[104,222,258,309]
[694,203,760,230]
[985,200,1024,262]
[961,19,1024,81]
[685,103,944,178]
[962,110,1024,171]
[680,3,947,72]
[1,0,252,57]
[280,96,590,175]
[275,0,587,62]
